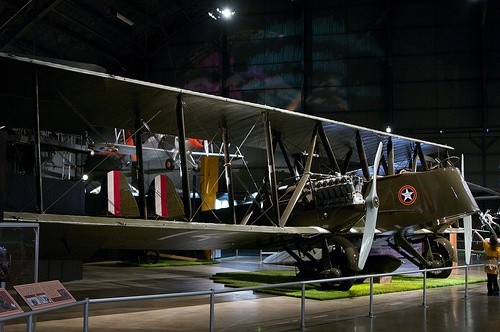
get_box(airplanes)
[0,52,479,292]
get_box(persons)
[475,230,500,296]
